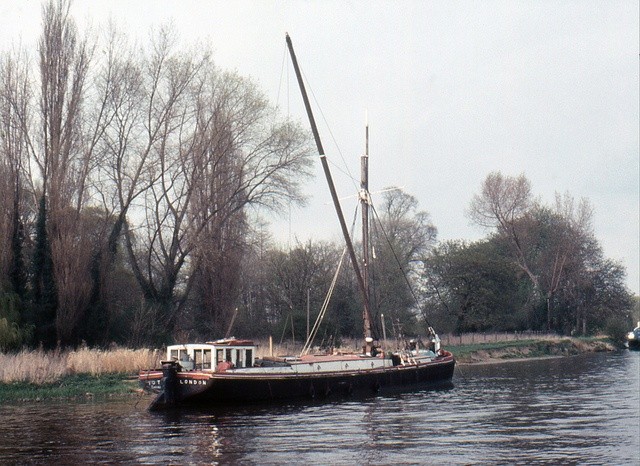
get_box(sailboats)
[139,31,456,411]
